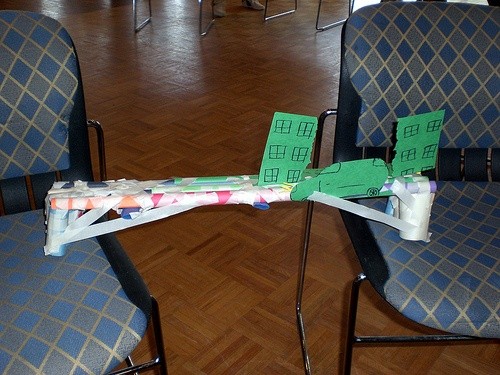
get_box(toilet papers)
[400,193,432,242]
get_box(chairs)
[296,1,500,375]
[0,10,169,375]
